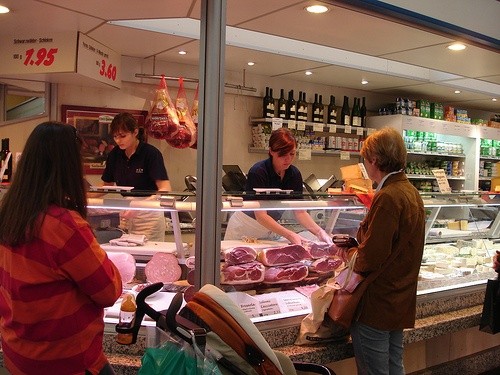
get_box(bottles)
[262,87,368,127]
[116,293,137,344]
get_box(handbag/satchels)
[139,328,222,375]
[478,278,500,336]
[293,249,363,346]
[324,279,366,329]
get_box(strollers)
[113,281,339,375]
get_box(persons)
[0,121,123,375]
[336,128,425,375]
[87,111,172,242]
[492,250,500,273]
[223,128,332,245]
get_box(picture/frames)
[62,105,149,175]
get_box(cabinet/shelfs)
[248,118,376,156]
[368,115,500,192]
